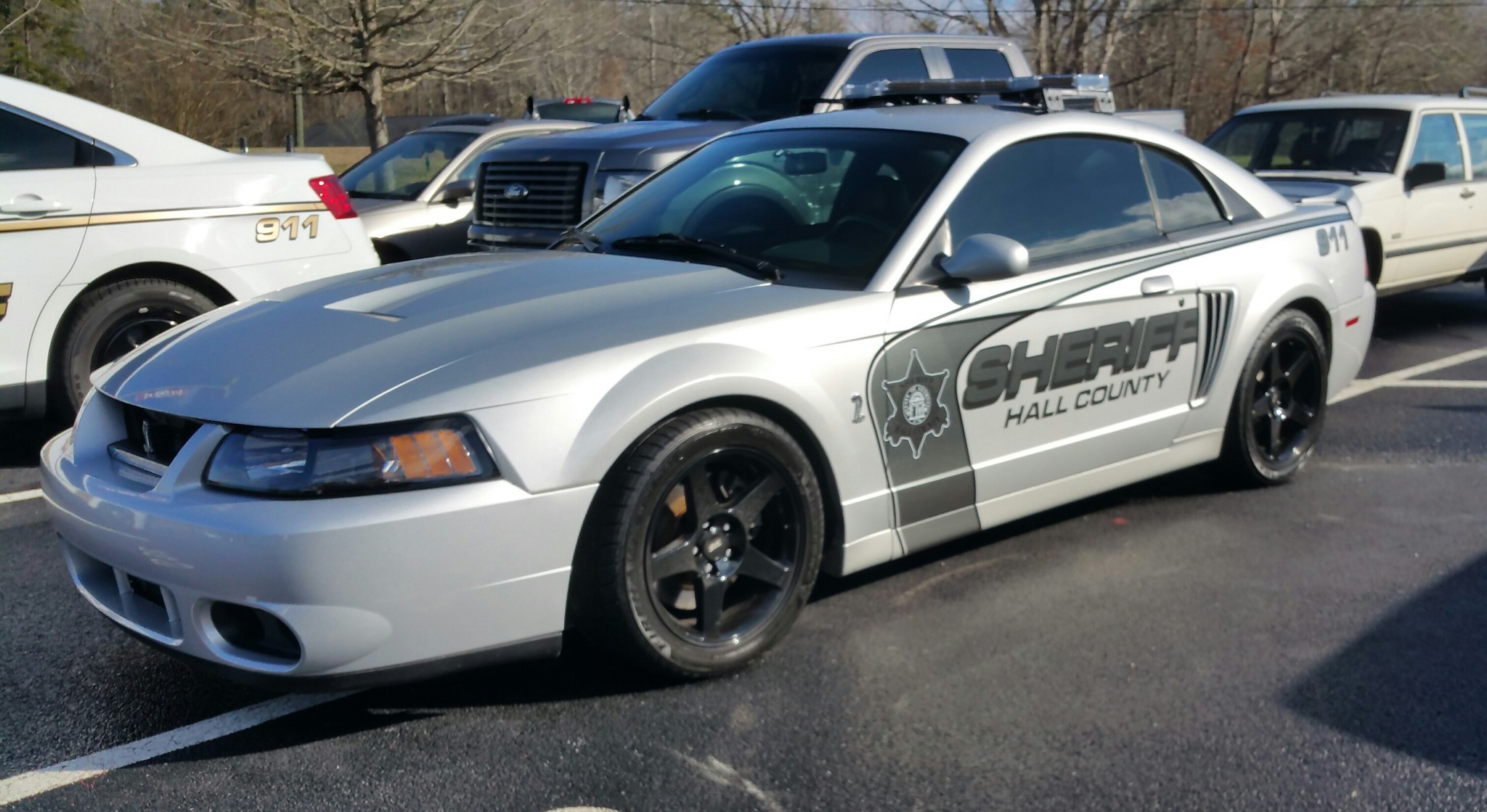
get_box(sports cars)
[36,67,1387,699]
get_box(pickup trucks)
[465,32,1191,253]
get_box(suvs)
[1188,82,1487,300]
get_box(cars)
[521,95,636,123]
[0,73,382,430]
[335,115,607,265]
